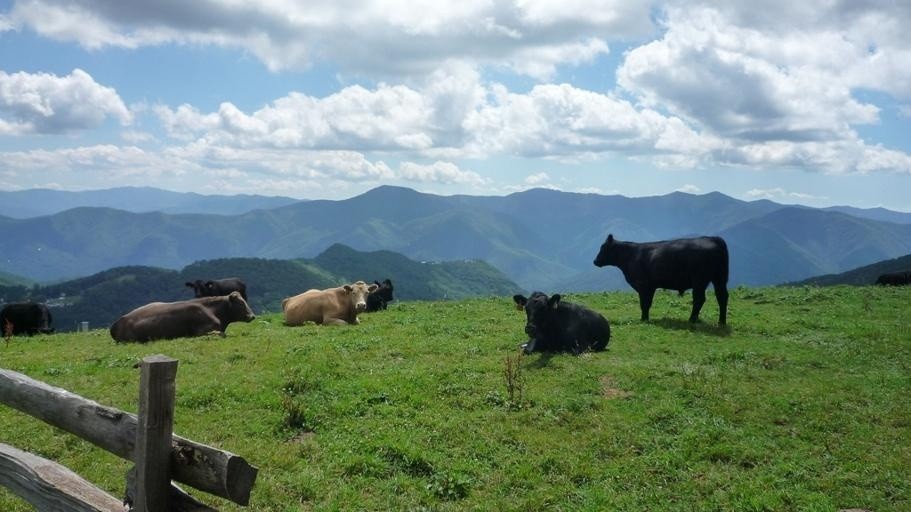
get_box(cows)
[110,290,256,345]
[593,234,730,328]
[511,290,611,356]
[185,278,248,303]
[874,270,911,287]
[364,278,394,312]
[0,302,54,337]
[281,281,379,327]
